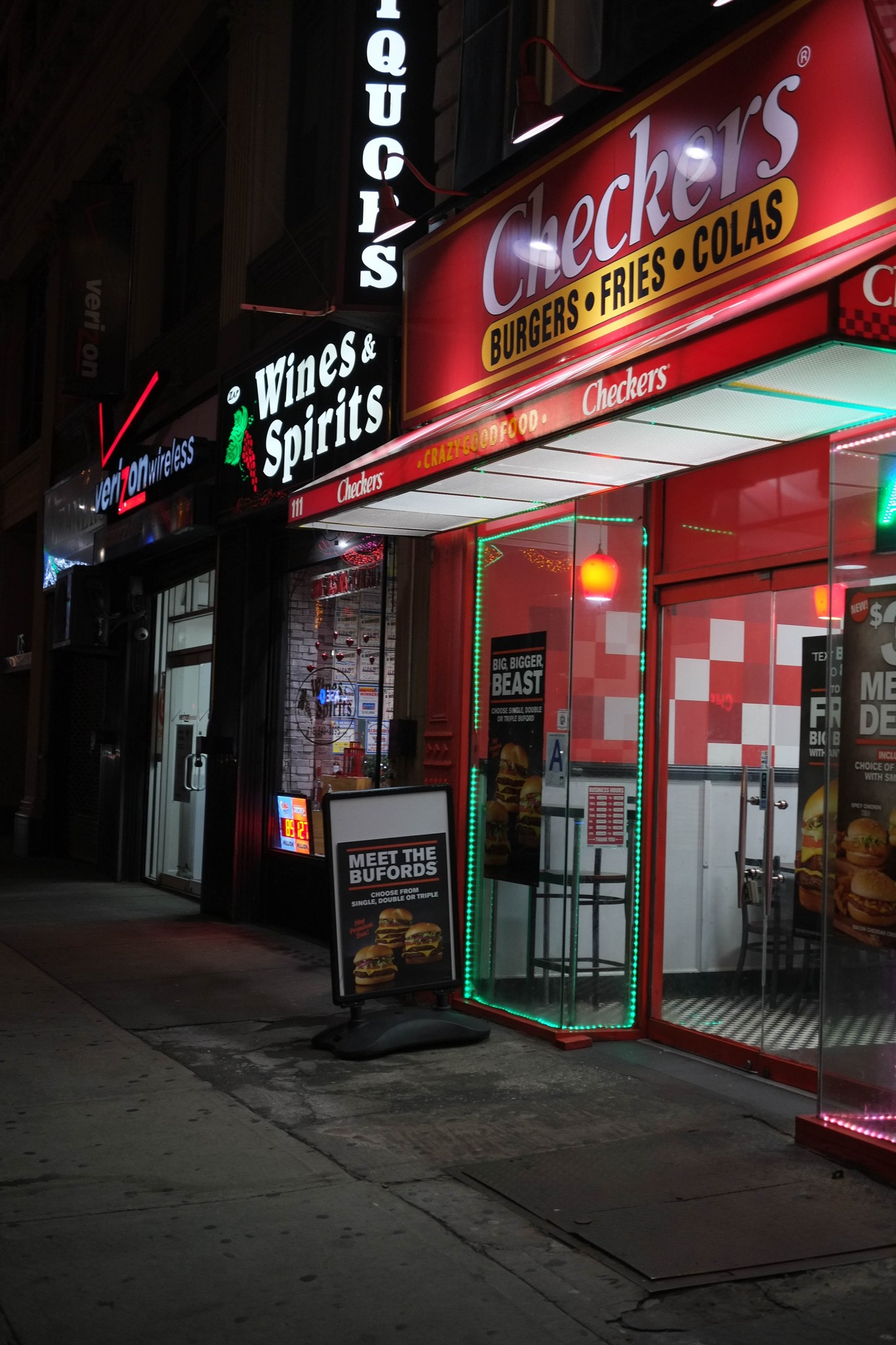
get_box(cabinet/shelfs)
[525,804,638,1026]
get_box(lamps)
[510,36,623,145]
[371,153,474,245]
[580,493,618,602]
[813,582,849,623]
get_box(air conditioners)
[51,565,101,651]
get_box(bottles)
[332,756,339,776]
[309,767,323,811]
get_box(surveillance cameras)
[133,627,148,640]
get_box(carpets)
[775,1042,895,1091]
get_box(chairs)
[734,850,875,1021]
